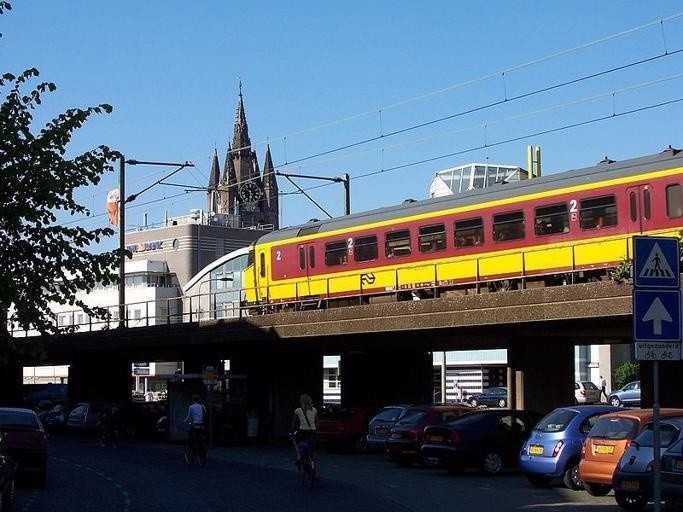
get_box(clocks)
[240,182,261,205]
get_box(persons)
[183,394,207,451]
[97,403,112,436]
[288,393,320,465]
[598,376,608,402]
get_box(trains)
[245,145,683,317]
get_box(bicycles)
[95,418,120,448]
[182,417,208,467]
[288,430,319,489]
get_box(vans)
[574,381,601,405]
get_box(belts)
[192,424,206,429]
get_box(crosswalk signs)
[632,237,680,289]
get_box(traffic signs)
[632,290,683,361]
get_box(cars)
[0,432,19,512]
[316,403,545,476]
[518,404,640,490]
[466,387,507,408]
[607,381,641,407]
[0,406,49,471]
[34,398,167,435]
[578,408,683,512]
